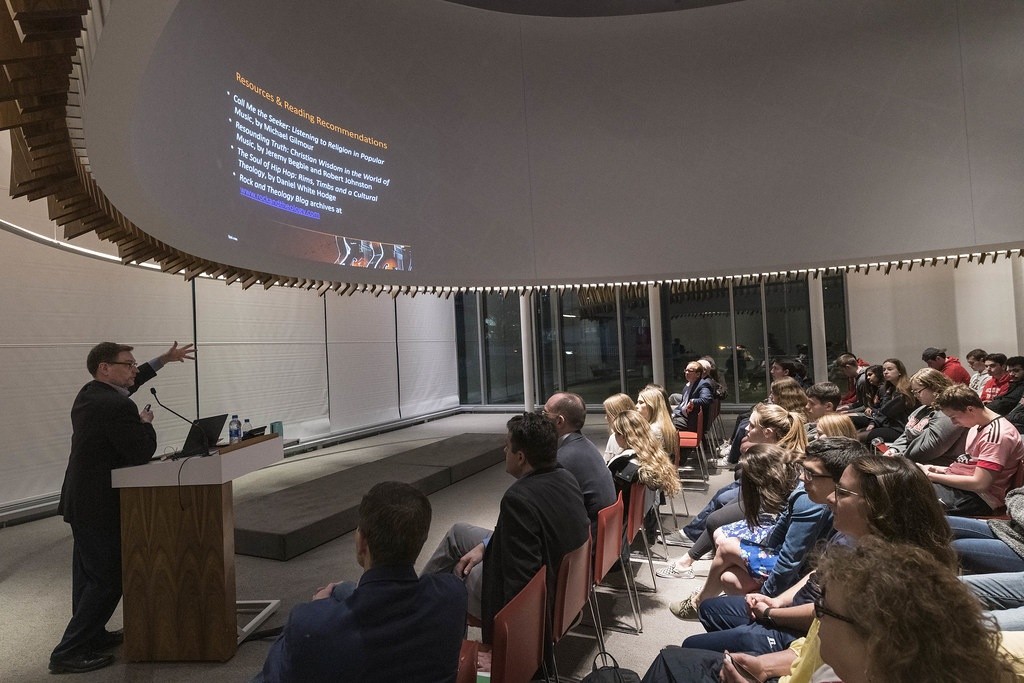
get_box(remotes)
[144,404,151,412]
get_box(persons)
[45,340,199,673]
[419,412,590,643]
[252,482,468,683]
[640,346,1024,683]
[602,383,683,530]
[541,391,617,555]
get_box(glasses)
[98,360,137,369]
[911,385,929,396]
[541,410,558,417]
[796,462,833,481]
[684,369,697,372]
[814,597,860,624]
[834,484,865,500]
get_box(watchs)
[763,607,777,627]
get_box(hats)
[922,348,947,361]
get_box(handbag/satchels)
[582,652,641,683]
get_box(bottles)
[229,415,242,445]
[242,419,252,432]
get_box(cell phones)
[724,653,762,683]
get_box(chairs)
[965,458,1024,519]
[457,399,728,683]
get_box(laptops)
[152,413,229,460]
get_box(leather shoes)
[48,653,113,671]
[90,628,124,650]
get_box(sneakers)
[656,563,694,578]
[708,456,738,468]
[657,529,695,547]
[720,445,733,457]
[669,596,700,620]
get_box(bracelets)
[926,472,931,477]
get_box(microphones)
[150,388,209,456]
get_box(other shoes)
[715,437,731,452]
